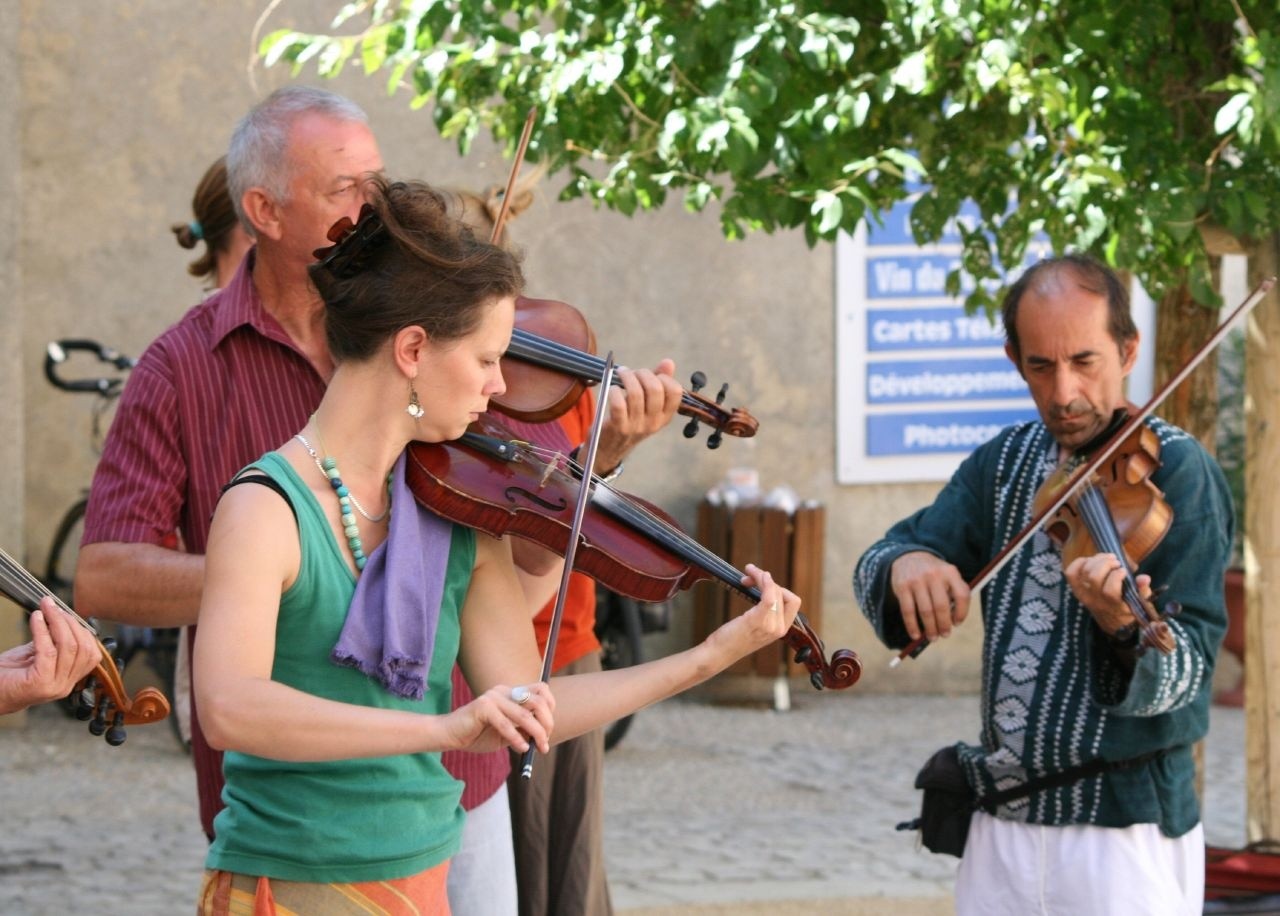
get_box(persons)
[174,156,261,303]
[851,252,1234,916]
[70,87,682,916]
[0,594,103,722]
[194,180,801,916]
[434,187,618,916]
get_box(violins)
[1030,406,1174,656]
[487,293,760,449]
[404,412,862,691]
[0,547,170,746]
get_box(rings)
[508,685,534,705]
[770,598,779,612]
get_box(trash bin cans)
[693,494,831,713]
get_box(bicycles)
[42,335,181,721]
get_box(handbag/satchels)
[894,745,1173,858]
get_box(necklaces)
[296,430,401,573]
